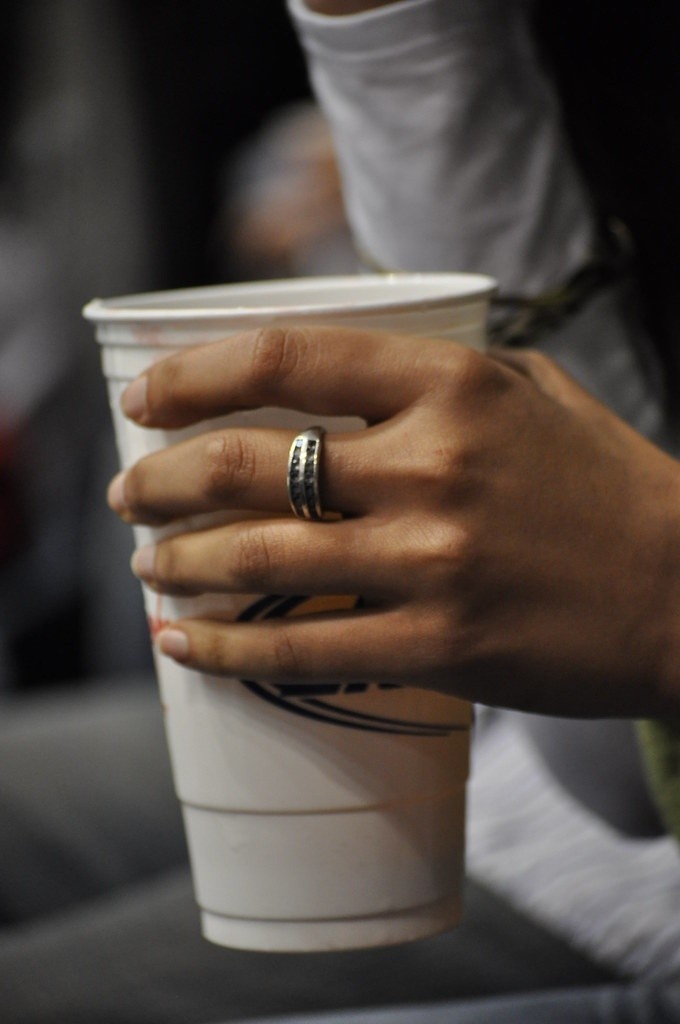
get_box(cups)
[82,273,504,954]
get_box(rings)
[285,424,325,523]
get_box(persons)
[0,0,680,1024]
